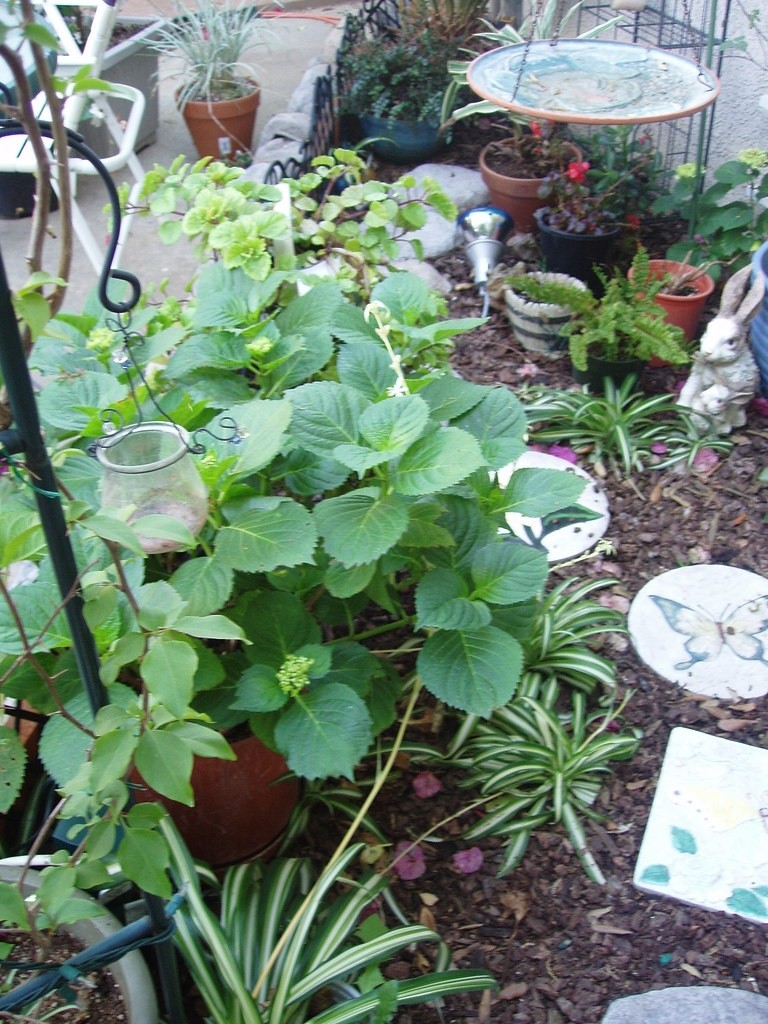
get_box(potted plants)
[0,0,126,219]
[436,0,629,142]
[0,147,604,1024]
[131,0,285,166]
[508,240,699,393]
[340,12,479,161]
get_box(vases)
[43,15,173,160]
[746,242,768,398]
[480,133,583,233]
[505,272,593,351]
[627,259,715,366]
[532,206,623,285]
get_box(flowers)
[512,118,666,235]
[658,147,768,290]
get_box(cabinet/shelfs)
[581,0,734,228]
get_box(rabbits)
[675,263,766,442]
